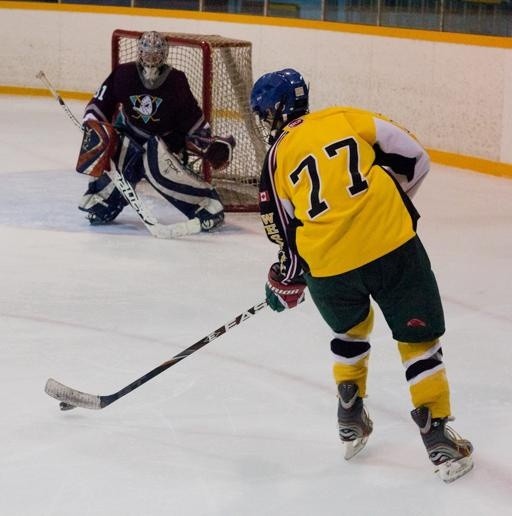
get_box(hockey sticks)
[35,72,203,239]
[46,299,270,410]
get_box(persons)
[75,29,237,233]
[246,65,476,469]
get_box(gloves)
[265,262,307,312]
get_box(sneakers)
[201,211,224,229]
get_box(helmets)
[136,31,169,80]
[250,69,308,113]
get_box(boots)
[337,381,373,441]
[411,406,473,466]
